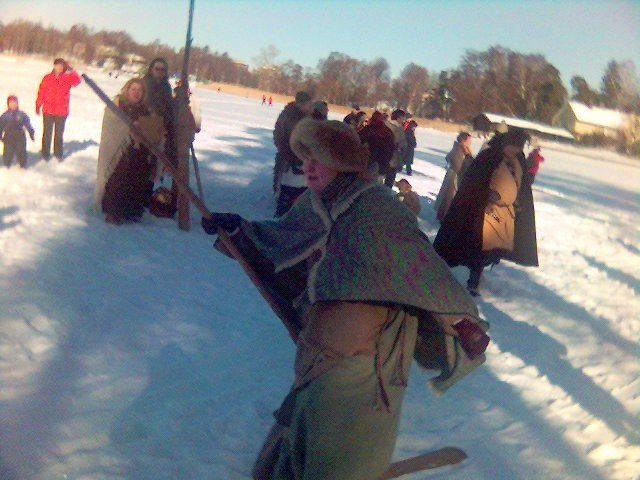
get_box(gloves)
[202,213,240,234]
[451,317,490,359]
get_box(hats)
[289,117,370,173]
[501,125,531,146]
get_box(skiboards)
[173,88,195,231]
[382,447,472,480]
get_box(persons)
[0,93,34,168]
[201,119,491,479]
[403,112,413,128]
[523,135,544,185]
[357,111,395,174]
[98,81,158,224]
[394,179,420,217]
[373,106,392,121]
[343,103,360,122]
[269,96,272,106]
[467,111,497,146]
[171,73,201,218]
[262,94,266,105]
[36,59,80,162]
[272,91,311,174]
[274,100,329,219]
[434,122,540,296]
[351,112,369,132]
[380,110,406,188]
[140,59,173,220]
[401,120,418,175]
[438,132,476,226]
